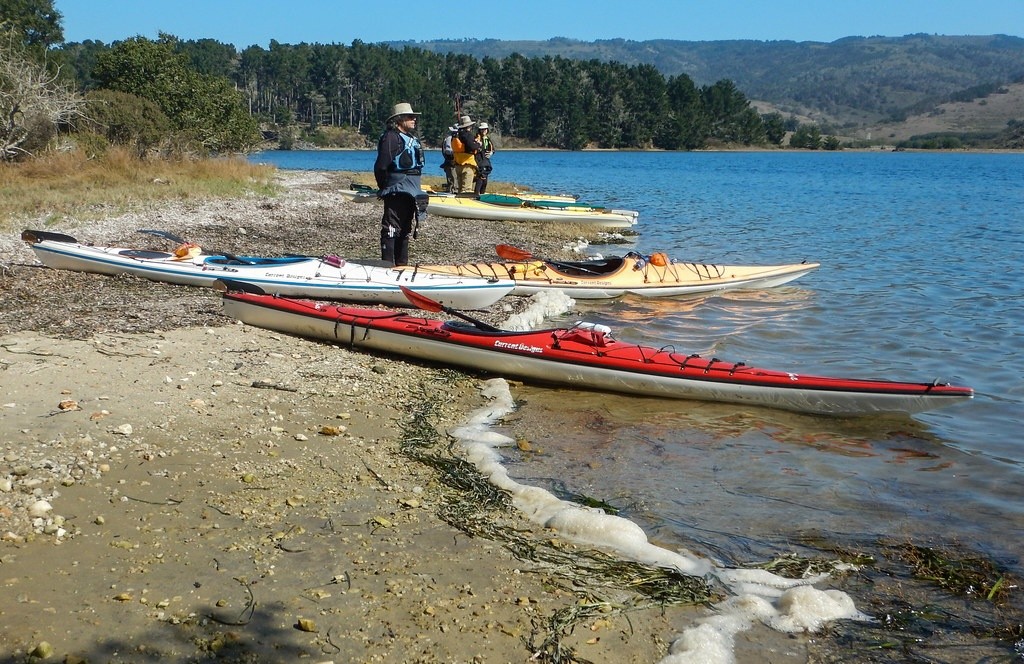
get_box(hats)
[478,122,488,129]
[449,123,459,132]
[385,103,422,125]
[457,116,476,128]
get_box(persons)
[440,116,495,193]
[375,103,425,264]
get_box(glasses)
[397,113,417,119]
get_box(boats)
[220,286,976,421]
[20,227,516,313]
[393,240,823,301]
[338,183,639,228]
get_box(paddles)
[397,284,503,334]
[137,228,258,265]
[493,243,604,277]
